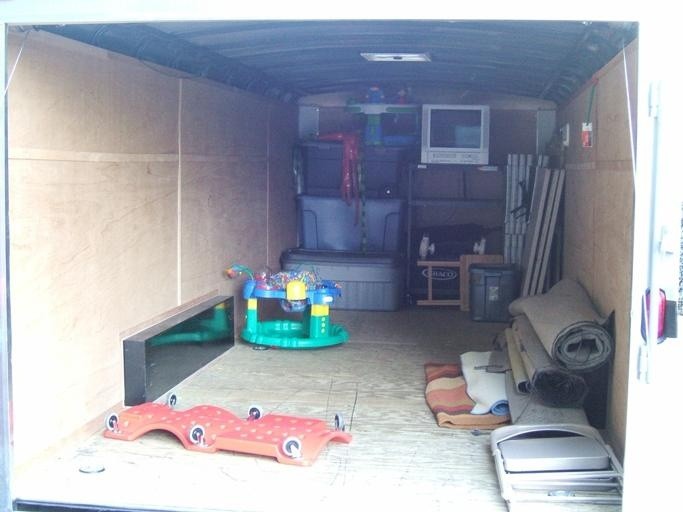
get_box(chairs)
[491,420,622,507]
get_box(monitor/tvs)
[421,104,489,166]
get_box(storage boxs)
[300,197,404,256]
[283,253,398,313]
[299,142,407,200]
